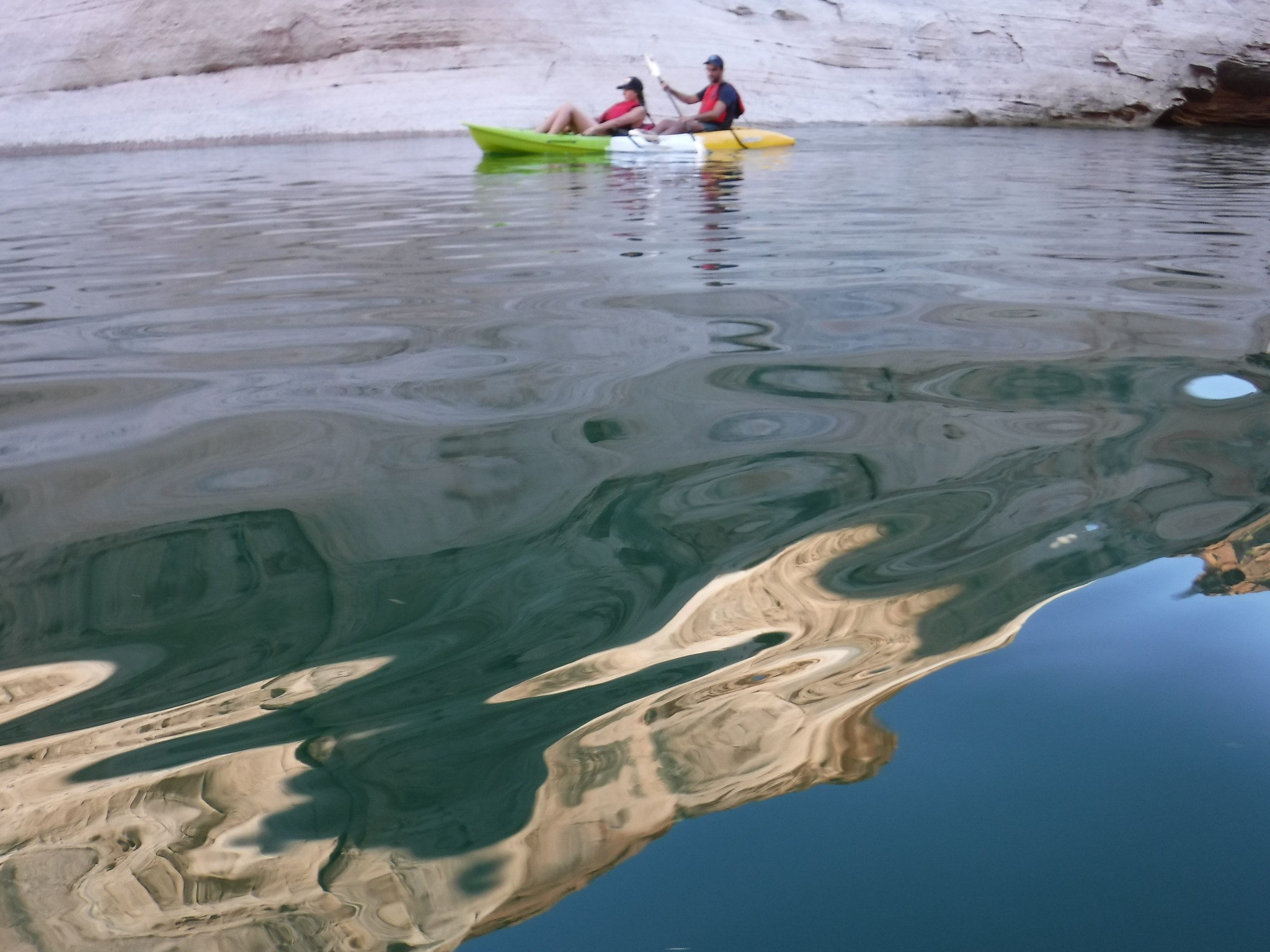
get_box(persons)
[649,55,744,135]
[533,76,650,136]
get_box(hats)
[703,54,723,69]
[616,76,643,92]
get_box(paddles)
[610,128,659,144]
[644,52,706,154]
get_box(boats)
[459,120,797,156]
